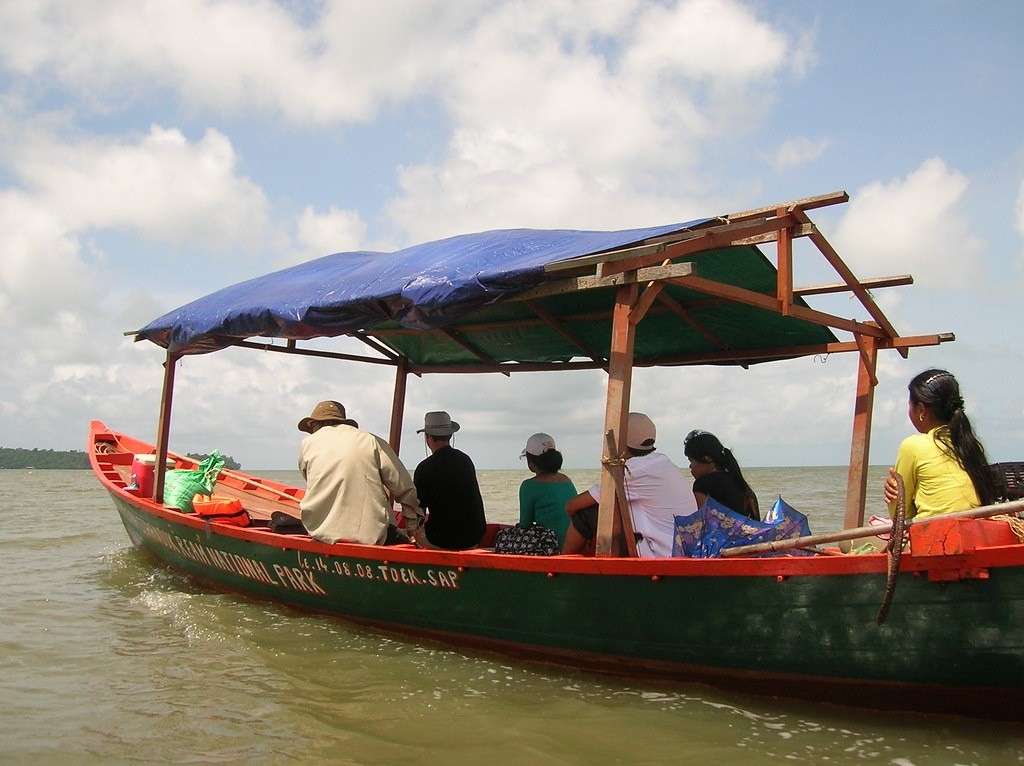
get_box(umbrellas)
[671,493,849,559]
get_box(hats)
[417,411,460,436]
[522,432,555,456]
[297,401,358,434]
[627,412,656,450]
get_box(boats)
[86,191,1024,721]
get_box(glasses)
[307,419,314,427]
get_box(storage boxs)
[130,454,175,497]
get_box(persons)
[559,413,699,558]
[837,369,1000,555]
[494,432,578,555]
[414,410,487,551]
[298,400,426,546]
[684,429,761,524]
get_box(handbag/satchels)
[495,526,560,556]
[868,515,910,540]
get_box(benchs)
[459,548,495,554]
[388,542,418,549]
